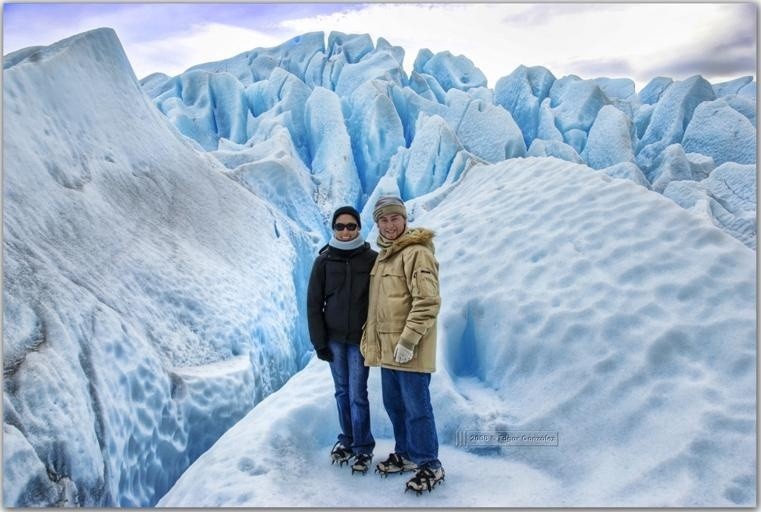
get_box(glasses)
[334,223,357,231]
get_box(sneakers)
[405,464,445,492]
[377,452,418,474]
[352,453,374,472]
[331,443,355,462]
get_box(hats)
[331,206,361,228]
[373,196,407,221]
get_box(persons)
[305,205,381,476]
[360,194,447,498]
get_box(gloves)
[316,348,333,362]
[394,344,413,364]
